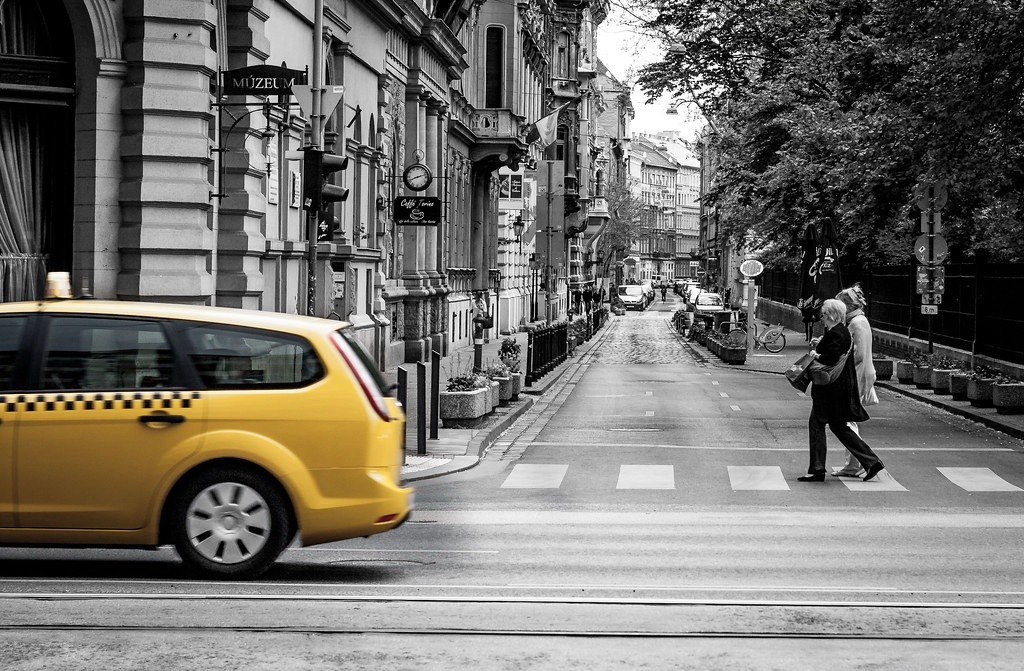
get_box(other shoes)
[798,473,825,482]
[831,469,858,477]
[855,464,865,476]
[863,459,884,481]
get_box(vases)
[440,389,486,429]
[477,386,492,421]
[489,381,500,413]
[491,377,514,408]
[509,372,521,402]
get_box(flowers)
[440,337,523,394]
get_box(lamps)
[592,249,605,266]
[508,215,525,242]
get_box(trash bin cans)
[714,310,732,333]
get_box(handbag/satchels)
[810,354,848,384]
[862,385,879,406]
[785,354,815,393]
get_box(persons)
[472,293,487,349]
[611,284,618,302]
[798,287,885,482]
[661,284,667,301]
[651,283,655,289]
[570,286,606,316]
[726,288,731,308]
[673,282,678,295]
[625,278,636,285]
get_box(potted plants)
[897,347,1024,416]
[871,351,893,381]
[707,330,747,366]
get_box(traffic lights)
[303,149,350,212]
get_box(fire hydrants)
[471,311,494,374]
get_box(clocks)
[403,149,432,191]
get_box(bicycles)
[727,311,786,354]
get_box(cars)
[640,279,706,304]
[2,271,418,581]
[618,284,645,312]
[686,288,708,311]
[691,292,728,322]
